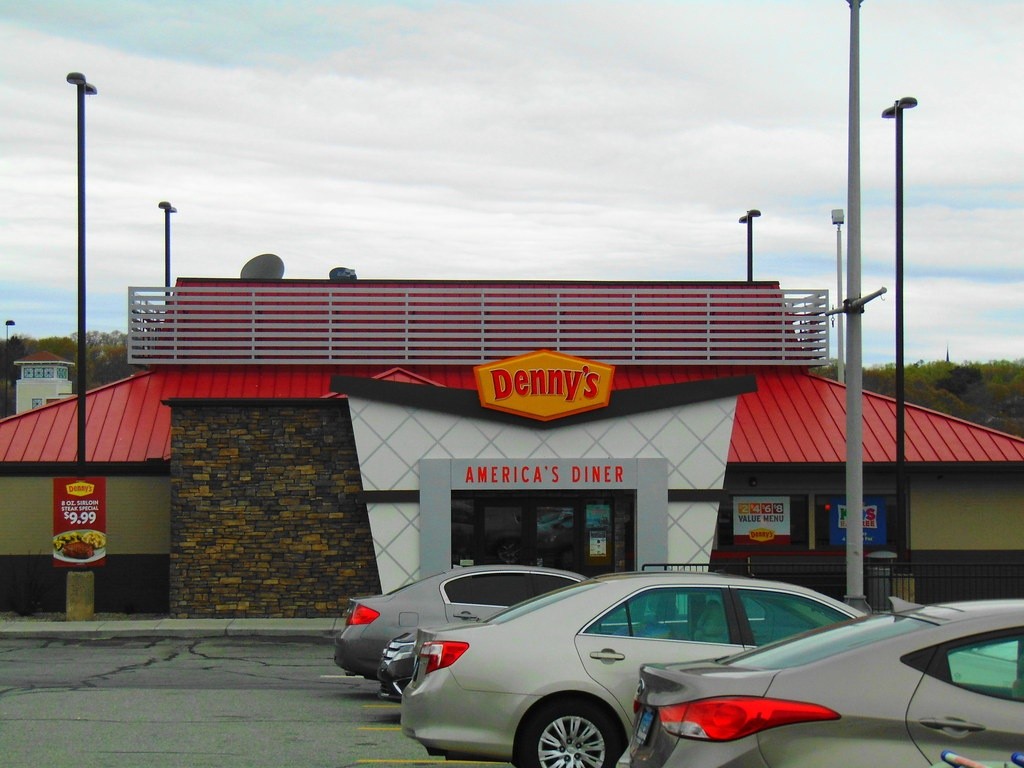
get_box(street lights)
[63,73,98,622]
[880,94,918,603]
[5,318,16,417]
[156,201,177,314]
[831,207,845,384]
[737,208,761,281]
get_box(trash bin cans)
[865,550,898,610]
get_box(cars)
[614,596,1024,768]
[400,571,1019,768]
[333,564,775,704]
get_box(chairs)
[693,600,730,644]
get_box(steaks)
[62,542,94,558]
